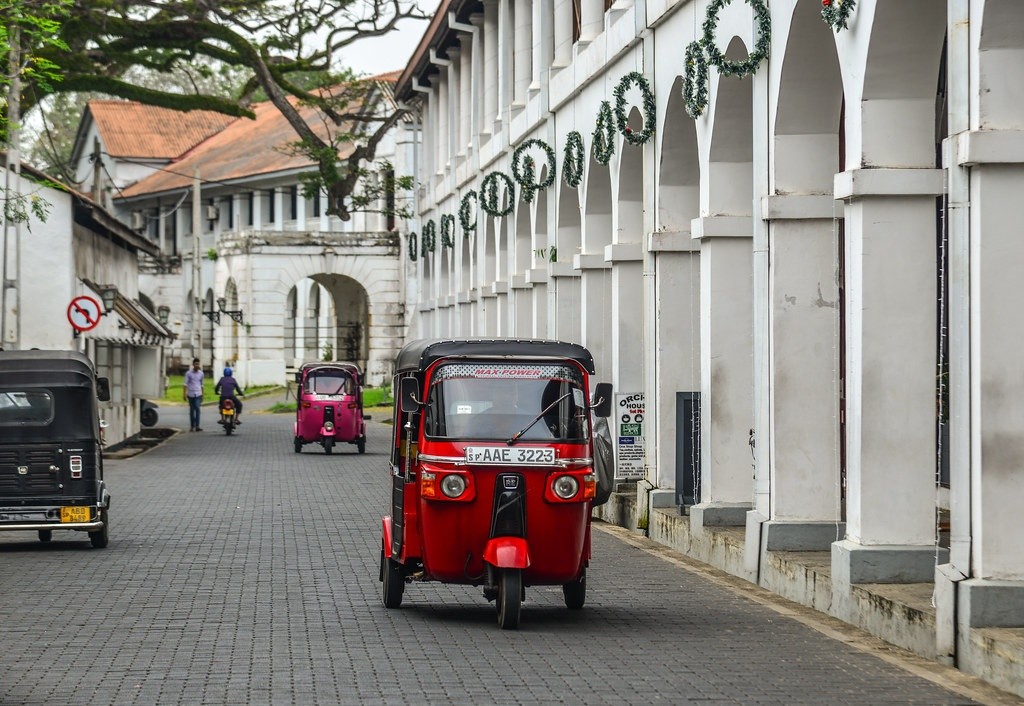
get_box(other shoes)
[217,420,225,424]
[190,428,203,432]
[235,420,242,424]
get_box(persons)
[461,379,549,441]
[214,368,246,426]
[183,357,206,432]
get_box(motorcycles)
[290,360,369,454]
[216,391,245,436]
[0,343,116,553]
[376,338,621,631]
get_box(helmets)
[224,368,232,377]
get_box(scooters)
[140,398,160,426]
